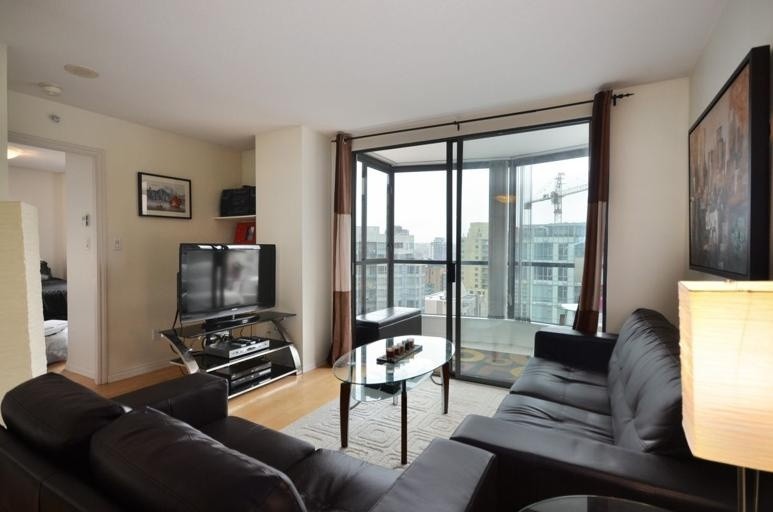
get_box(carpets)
[431,346,532,388]
[277,368,511,474]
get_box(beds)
[40,260,66,320]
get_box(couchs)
[449,307,744,510]
[0,373,495,511]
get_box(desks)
[512,493,675,512]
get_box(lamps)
[677,275,771,512]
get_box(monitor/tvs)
[179,242,276,331]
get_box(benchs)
[356,306,421,346]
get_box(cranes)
[524,172,588,223]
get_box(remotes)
[231,343,246,347]
[238,338,256,345]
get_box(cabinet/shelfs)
[156,311,301,397]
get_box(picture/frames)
[137,171,192,219]
[687,44,772,281]
